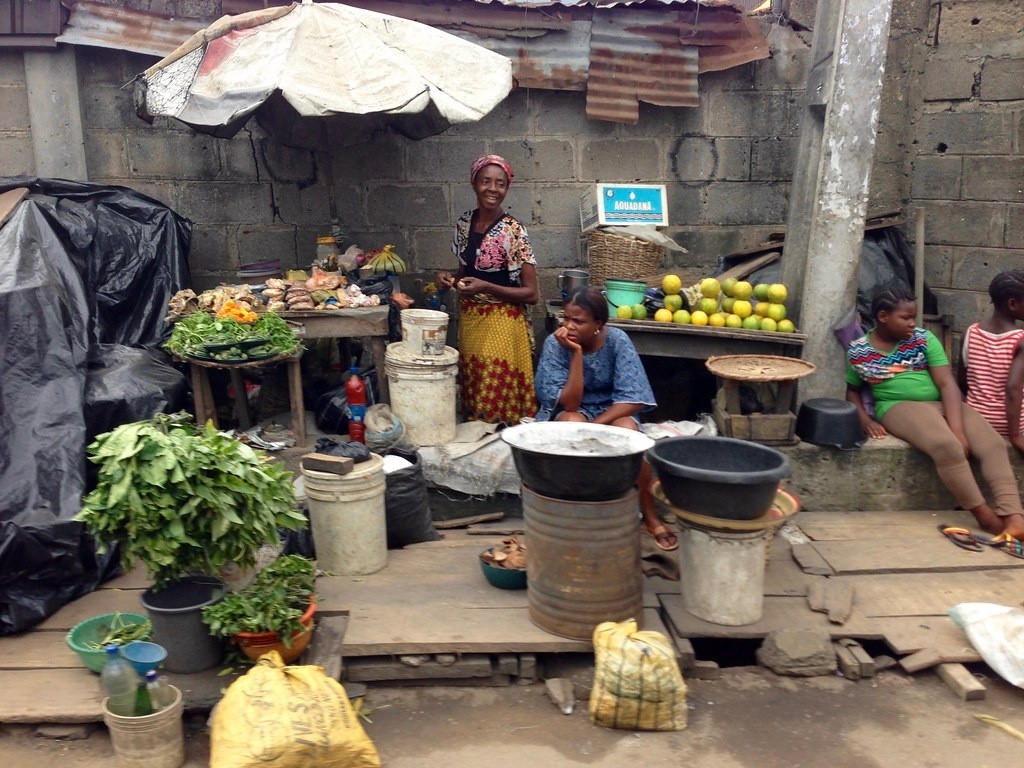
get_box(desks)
[175,306,388,431]
[605,319,806,406]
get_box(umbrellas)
[121,0,514,238]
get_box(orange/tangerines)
[655,274,795,333]
[617,304,647,320]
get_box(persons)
[846,288,1024,542]
[437,154,539,426]
[956,268,1024,455]
[532,286,679,551]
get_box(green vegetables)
[202,554,333,649]
[70,413,308,594]
[163,309,308,360]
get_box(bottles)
[102,645,172,716]
[345,368,367,442]
[316,237,341,271]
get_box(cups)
[298,451,389,575]
[556,269,589,295]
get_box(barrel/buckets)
[520,480,643,640]
[675,515,762,626]
[144,575,228,675]
[399,307,450,355]
[383,344,459,446]
[605,281,646,317]
[101,685,186,768]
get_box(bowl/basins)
[646,435,789,520]
[479,548,526,588]
[500,421,656,501]
[238,258,282,285]
[795,398,869,452]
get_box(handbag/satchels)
[318,368,379,435]
[643,287,691,317]
[367,245,406,273]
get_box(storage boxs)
[580,182,668,233]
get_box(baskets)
[586,231,664,291]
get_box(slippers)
[643,524,679,550]
[936,525,983,552]
[970,532,1024,558]
